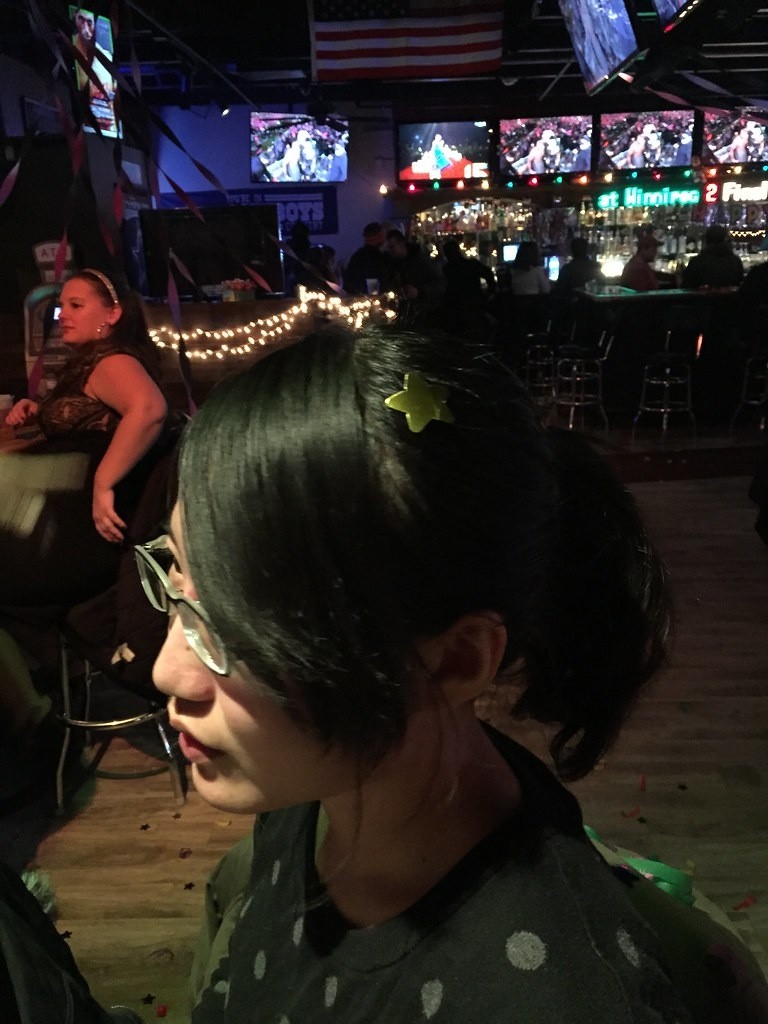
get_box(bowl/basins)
[0,396,14,410]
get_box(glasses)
[133,532,246,676]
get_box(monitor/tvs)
[495,113,593,176]
[556,0,652,95]
[594,107,697,177]
[249,111,352,182]
[695,97,768,172]
[652,0,705,35]
[392,116,492,186]
[138,204,283,297]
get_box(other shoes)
[9,693,54,752]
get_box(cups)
[367,278,378,295]
[586,281,620,294]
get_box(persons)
[133,328,768,1023]
[681,225,744,287]
[0,270,169,760]
[192,221,494,334]
[702,115,768,162]
[510,241,550,295]
[600,114,695,169]
[619,237,673,291]
[704,262,768,433]
[431,134,449,155]
[72,8,115,130]
[571,0,634,75]
[500,118,592,174]
[558,238,607,287]
[251,129,347,181]
[0,859,141,1024]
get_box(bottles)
[586,230,702,254]
[580,201,726,226]
[421,204,504,232]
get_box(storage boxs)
[221,289,255,302]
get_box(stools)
[43,598,193,813]
[723,348,768,438]
[518,330,559,403]
[630,351,700,442]
[543,347,611,434]
